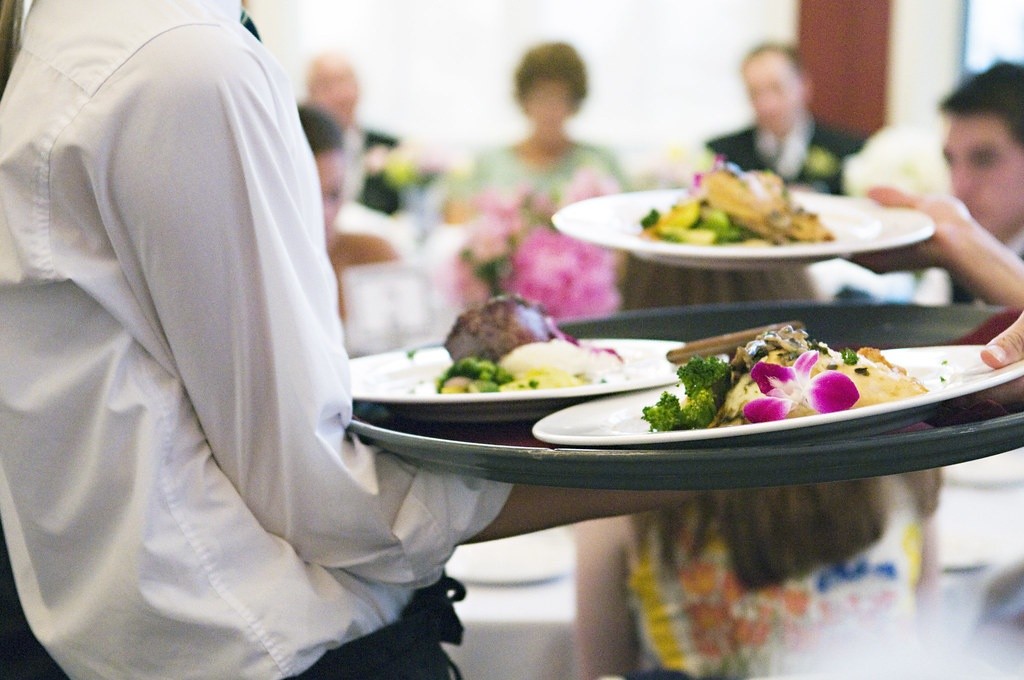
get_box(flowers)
[743,349,860,424]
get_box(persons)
[297,46,452,359]
[840,184,1024,369]
[472,42,636,194]
[936,55,1024,304]
[703,41,865,195]
[574,252,943,680]
[1,1,711,680]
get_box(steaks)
[446,293,552,361]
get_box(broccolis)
[437,357,514,395]
[641,353,734,430]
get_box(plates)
[345,337,729,452]
[548,189,937,274]
[530,344,1024,448]
